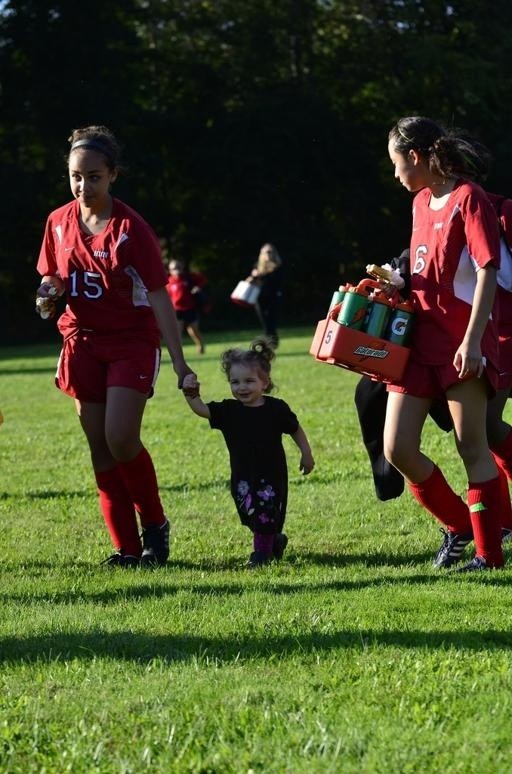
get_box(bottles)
[326,282,416,348]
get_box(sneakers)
[433,527,512,574]
[273,533,288,560]
[107,551,141,570]
[240,551,273,568]
[142,518,169,564]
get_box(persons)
[244,240,287,350]
[375,114,507,571]
[29,123,204,567]
[164,257,206,356]
[447,131,512,548]
[180,342,317,561]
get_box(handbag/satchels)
[230,281,260,305]
